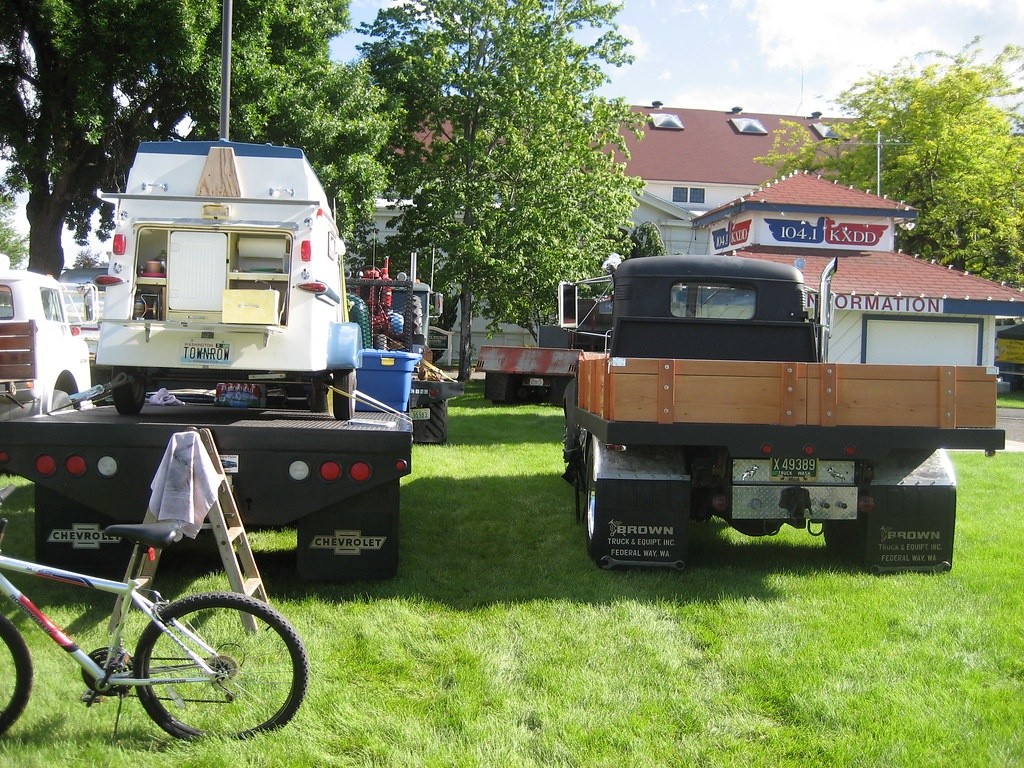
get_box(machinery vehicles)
[98,0,368,417]
[354,255,466,445]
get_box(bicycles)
[1,483,308,743]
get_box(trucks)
[1,405,415,583]
[474,297,620,404]
[554,253,1007,576]
[1,269,98,418]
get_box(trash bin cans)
[356,349,423,412]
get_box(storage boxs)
[222,289,282,324]
[354,349,423,413]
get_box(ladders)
[107,427,276,656]
